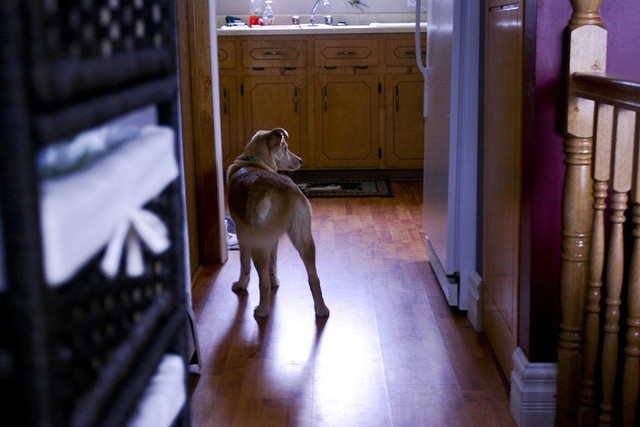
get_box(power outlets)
[408,0,416,7]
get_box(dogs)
[227,128,329,317]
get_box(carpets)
[295,178,394,199]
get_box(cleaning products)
[262,1,274,26]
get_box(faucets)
[310,0,330,26]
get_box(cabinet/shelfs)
[384,39,425,169]
[217,41,239,171]
[242,39,383,171]
